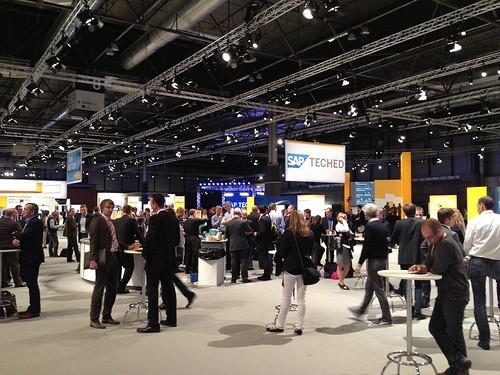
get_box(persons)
[114,205,144,294]
[348,204,465,319]
[409,219,472,375]
[266,210,314,335]
[137,194,177,333]
[160,209,197,309]
[254,206,272,281]
[111,201,338,276]
[464,196,500,350]
[335,212,354,290]
[63,204,100,262]
[348,203,392,325]
[47,211,61,257]
[12,203,45,319]
[0,205,49,288]
[226,208,252,283]
[89,199,120,329]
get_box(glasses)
[422,232,436,241]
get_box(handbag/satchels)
[60,248,73,257]
[324,259,355,279]
[84,248,106,269]
[302,265,322,285]
[0,290,18,317]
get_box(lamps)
[348,25,370,41]
[404,83,429,105]
[265,85,297,105]
[0,81,122,178]
[76,4,104,33]
[55,35,71,49]
[222,33,262,83]
[442,28,467,54]
[44,55,61,71]
[231,106,243,117]
[142,71,198,109]
[124,122,238,170]
[107,42,118,55]
[304,112,317,127]
[301,0,346,21]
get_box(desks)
[0,248,22,320]
[122,249,163,324]
[200,237,228,279]
[352,236,369,288]
[377,269,442,375]
[322,233,337,263]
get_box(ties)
[107,219,118,248]
[18,215,21,221]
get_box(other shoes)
[231,279,252,283]
[372,318,392,325]
[50,255,60,257]
[102,318,120,324]
[248,267,254,270]
[257,274,271,280]
[67,260,75,262]
[338,283,350,290]
[1,283,25,288]
[349,307,361,317]
[160,302,166,310]
[116,289,129,294]
[437,358,472,375]
[266,327,284,332]
[294,328,302,335]
[186,292,195,307]
[412,312,426,319]
[478,341,490,350]
[90,321,106,329]
[17,309,40,319]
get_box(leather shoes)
[160,320,177,327]
[137,327,161,333]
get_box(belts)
[110,252,117,255]
